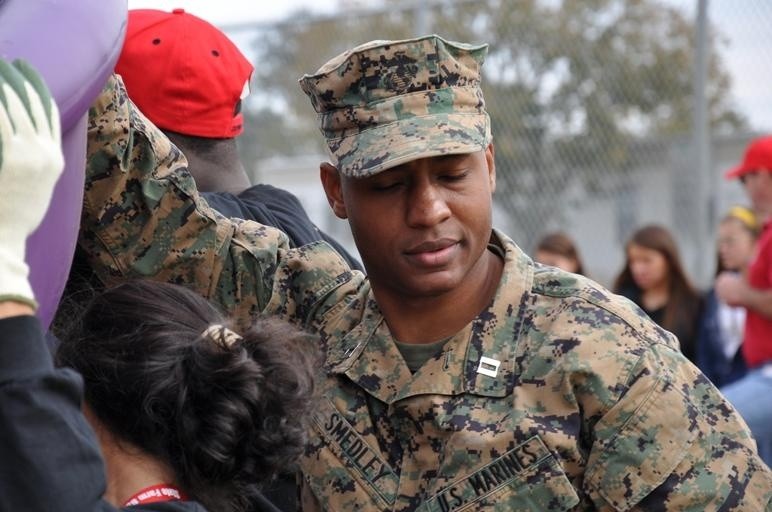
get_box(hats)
[726,136,772,180]
[112,8,254,139]
[297,35,491,180]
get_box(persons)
[1,59,324,511]
[716,133,771,469]
[610,225,704,367]
[534,232,586,278]
[692,206,763,390]
[114,9,366,278]
[79,34,770,511]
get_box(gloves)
[0,57,66,308]
